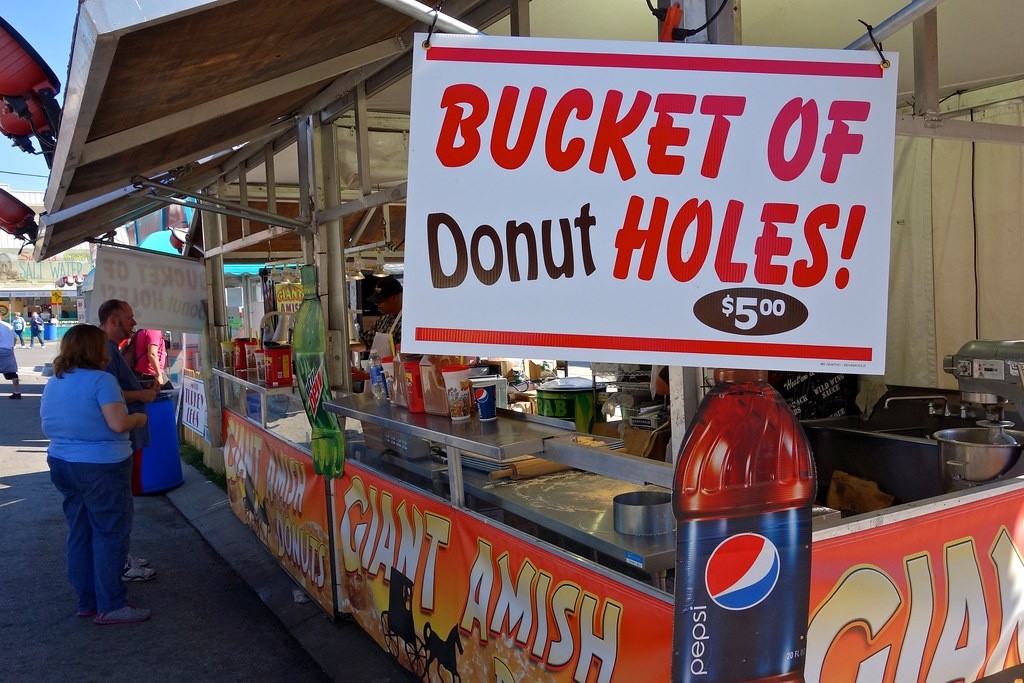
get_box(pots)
[933,428,1024,494]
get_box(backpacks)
[120,329,160,372]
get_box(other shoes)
[14,346,16,349]
[41,346,46,349]
[28,346,31,349]
[21,344,26,348]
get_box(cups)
[254,350,265,382]
[472,382,497,422]
[441,363,471,421]
[220,341,235,369]
[245,342,257,370]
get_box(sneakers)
[76,610,96,616]
[126,554,149,568]
[93,605,151,624]
[121,560,158,581]
[9,392,22,399]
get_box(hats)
[366,277,403,304]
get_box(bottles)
[671,370,818,683]
[292,265,344,478]
[369,349,388,406]
[393,344,455,417]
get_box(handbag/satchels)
[38,324,45,331]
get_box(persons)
[12,312,26,348]
[98,300,159,582]
[347,278,403,352]
[28,312,46,349]
[137,329,165,389]
[0,313,21,399]
[51,315,59,324]
[655,366,669,395]
[39,325,150,623]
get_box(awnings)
[34,0,518,265]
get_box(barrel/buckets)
[234,337,257,369]
[263,341,292,384]
[44,323,57,340]
[141,393,184,492]
